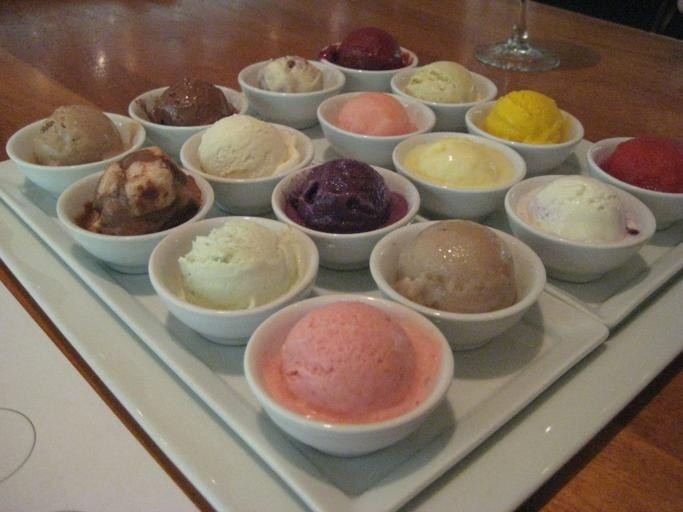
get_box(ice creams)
[31,26,683,426]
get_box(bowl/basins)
[371,220,547,353]
[9,111,146,202]
[181,123,314,209]
[52,169,214,277]
[147,214,321,346]
[319,42,421,92]
[391,131,528,218]
[128,80,248,161]
[314,95,438,164]
[466,104,585,169]
[270,164,421,271]
[503,172,656,282]
[390,66,497,128]
[236,59,343,130]
[241,290,456,458]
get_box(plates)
[1,144,612,512]
[299,104,683,326]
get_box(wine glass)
[473,1,560,74]
